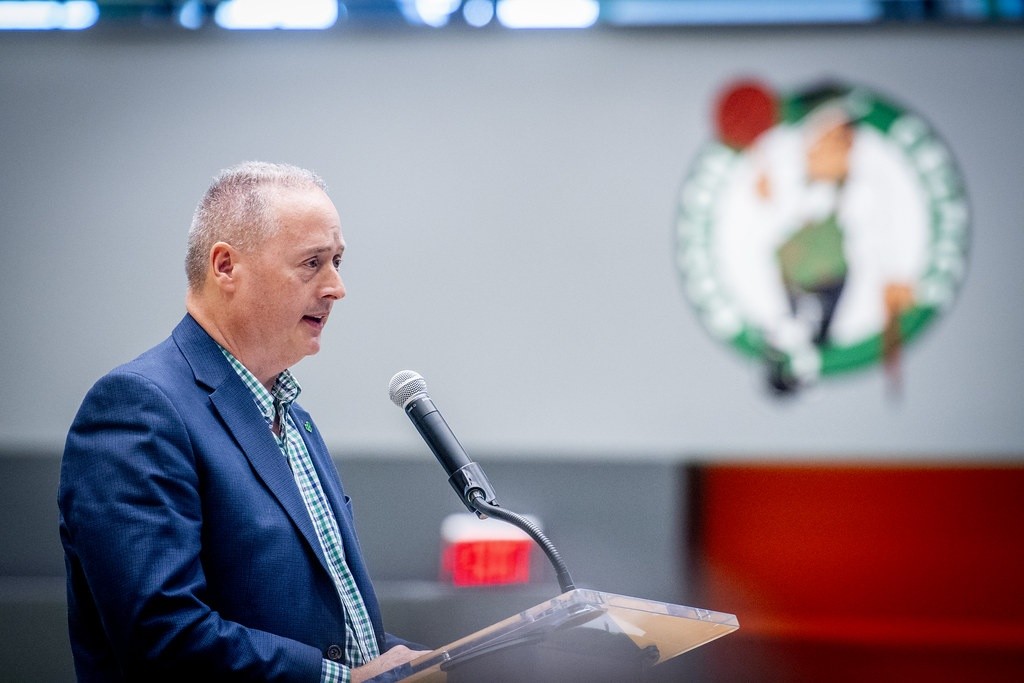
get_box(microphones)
[387,369,499,519]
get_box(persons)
[57,159,446,683]
